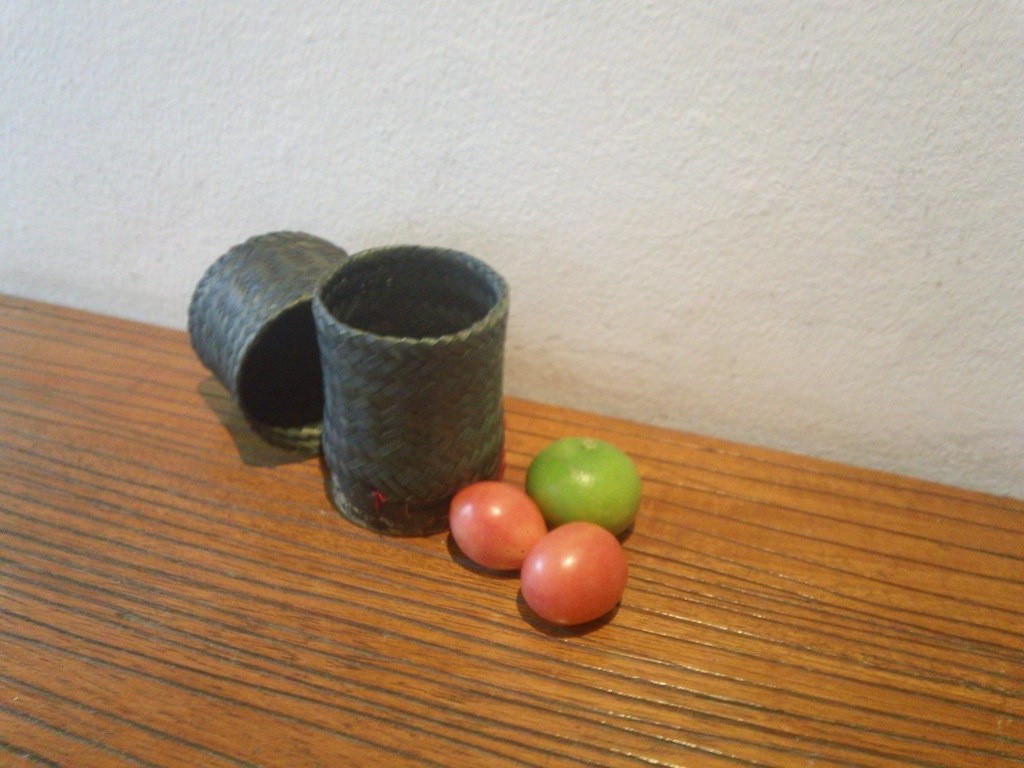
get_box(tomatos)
[448,480,548,570]
[521,521,628,625]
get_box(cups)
[310,243,508,537]
[188,231,349,454]
